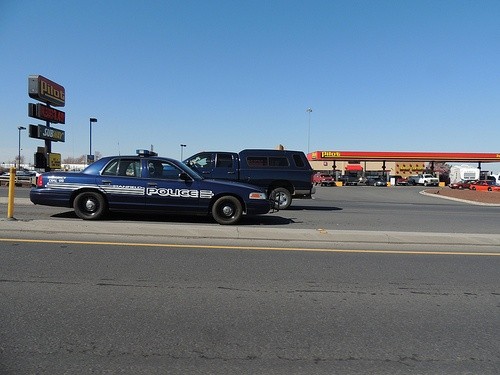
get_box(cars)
[367,178,386,186]
[339,174,360,185]
[29,149,273,225]
[450,180,500,192]
[396,178,409,185]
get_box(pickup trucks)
[408,174,439,186]
[177,149,315,210]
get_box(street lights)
[180,145,186,162]
[89,118,97,155]
[306,107,312,153]
[18,126,27,170]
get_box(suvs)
[314,173,335,186]
